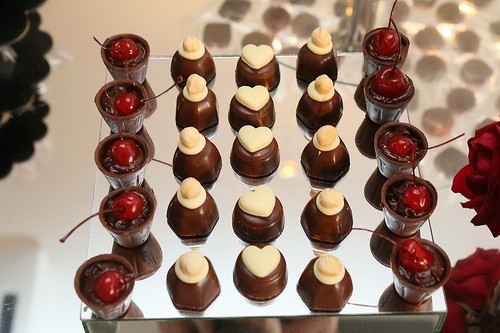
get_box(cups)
[100,33,150,84]
[370,219,420,267]
[141,78,157,118]
[95,79,148,133]
[94,132,151,190]
[389,238,451,305]
[380,174,438,238]
[364,166,388,211]
[74,255,136,319]
[107,125,155,163]
[362,71,415,128]
[361,27,410,76]
[353,74,368,113]
[99,185,156,249]
[355,115,381,158]
[378,282,434,313]
[374,122,429,180]
[108,179,155,194]
[111,232,162,280]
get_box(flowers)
[450,121,500,238]
[445,248,500,333]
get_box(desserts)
[175,74,220,132]
[228,85,276,132]
[300,125,351,187]
[300,187,353,244]
[167,252,220,312]
[169,34,216,87]
[166,177,220,239]
[235,43,281,93]
[297,254,354,311]
[296,74,343,131]
[60,33,182,321]
[296,27,338,82]
[230,125,281,178]
[232,185,284,243]
[173,127,222,184]
[344,0,465,303]
[233,243,288,300]
